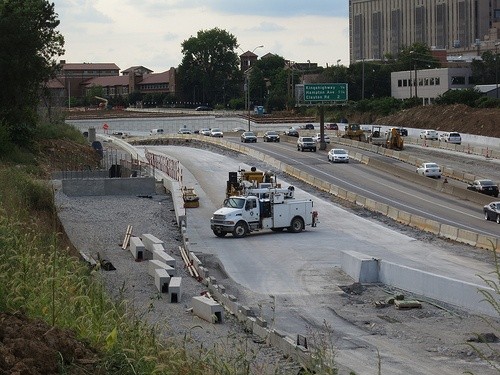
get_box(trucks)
[210,183,314,239]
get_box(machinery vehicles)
[384,128,404,152]
[342,122,365,142]
[366,126,386,146]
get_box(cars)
[328,148,349,163]
[482,202,500,224]
[440,132,462,145]
[195,106,212,111]
[400,128,408,136]
[420,130,438,140]
[467,178,499,198]
[240,132,257,143]
[149,123,338,138]
[415,162,442,178]
[263,131,280,143]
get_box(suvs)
[297,137,316,152]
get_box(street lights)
[409,51,415,98]
[336,59,341,83]
[360,57,366,100]
[307,60,310,69]
[236,45,264,132]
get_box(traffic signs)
[303,83,348,101]
